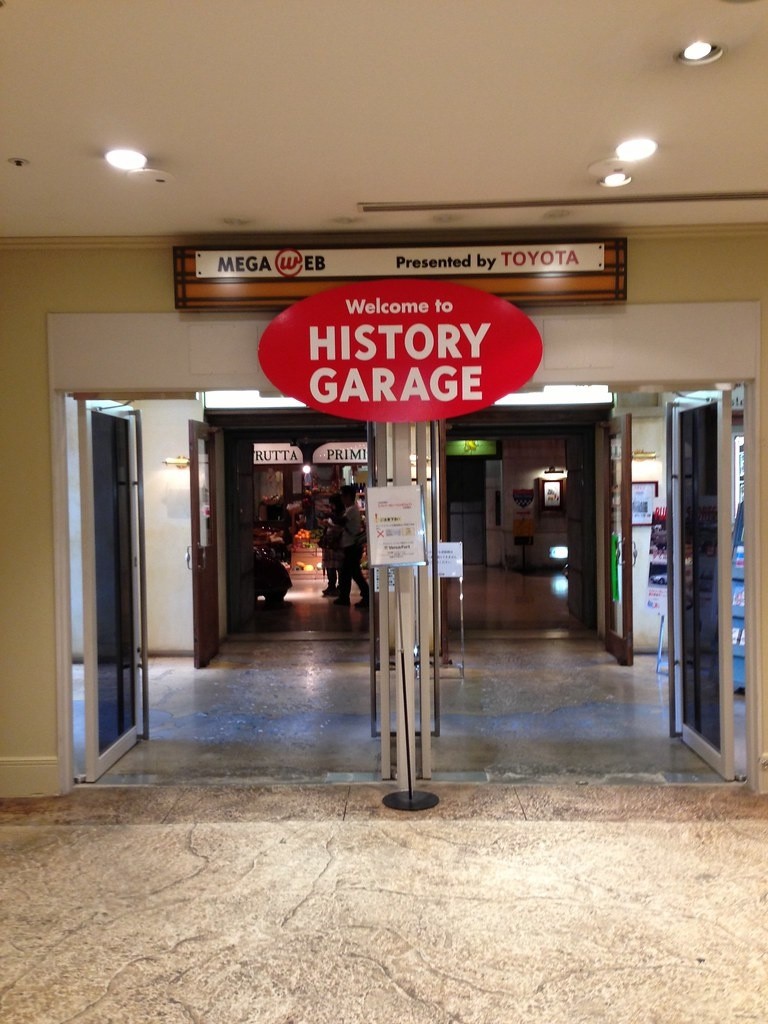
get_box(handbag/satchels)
[318,534,340,550]
[353,530,367,546]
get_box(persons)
[334,485,369,607]
[302,490,315,530]
[271,506,290,558]
[318,495,344,596]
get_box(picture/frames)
[632,480,660,526]
[537,477,566,515]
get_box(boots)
[333,591,350,605]
[322,582,341,596]
[354,591,370,608]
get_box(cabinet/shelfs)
[291,493,364,577]
